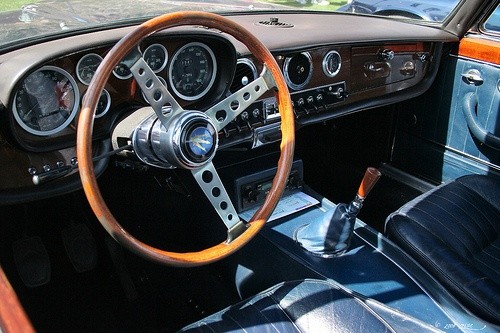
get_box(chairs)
[177,279,397,333]
[384,173,500,326]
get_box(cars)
[334,0,500,33]
